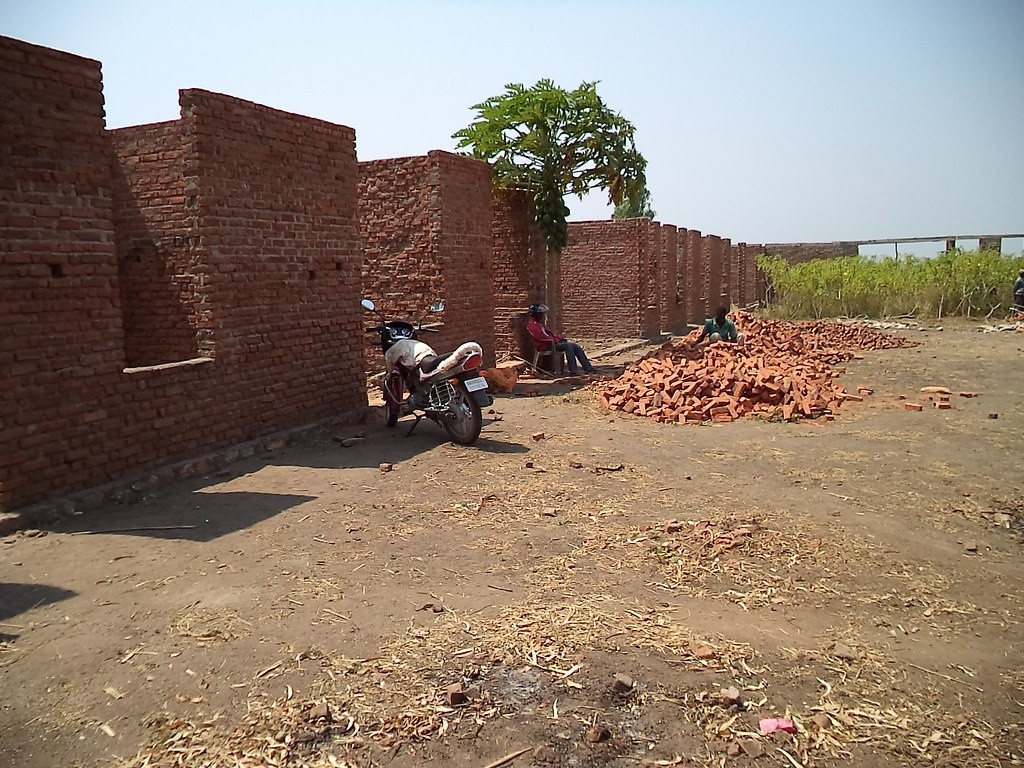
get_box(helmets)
[529,304,549,316]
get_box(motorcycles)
[360,296,494,445]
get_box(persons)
[527,304,598,375]
[691,303,737,348]
[1012,269,1024,312]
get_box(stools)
[527,326,566,375]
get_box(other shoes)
[570,374,578,377]
[584,370,601,375]
